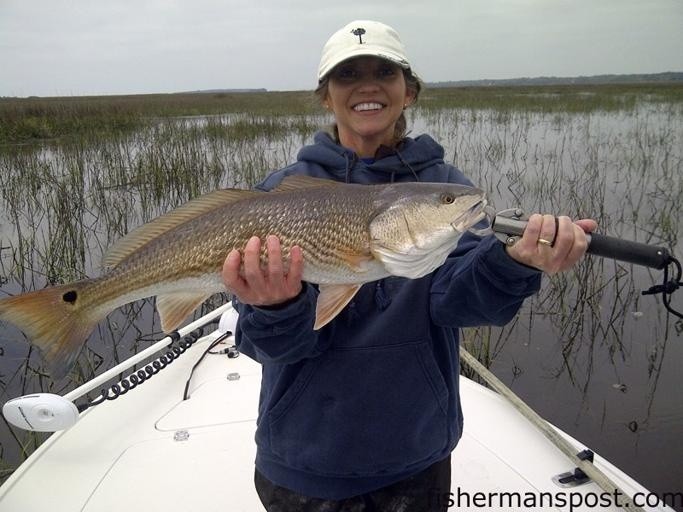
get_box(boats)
[0,298,683,512]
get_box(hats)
[318,20,408,83]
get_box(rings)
[538,239,552,245]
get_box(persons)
[223,20,597,511]
